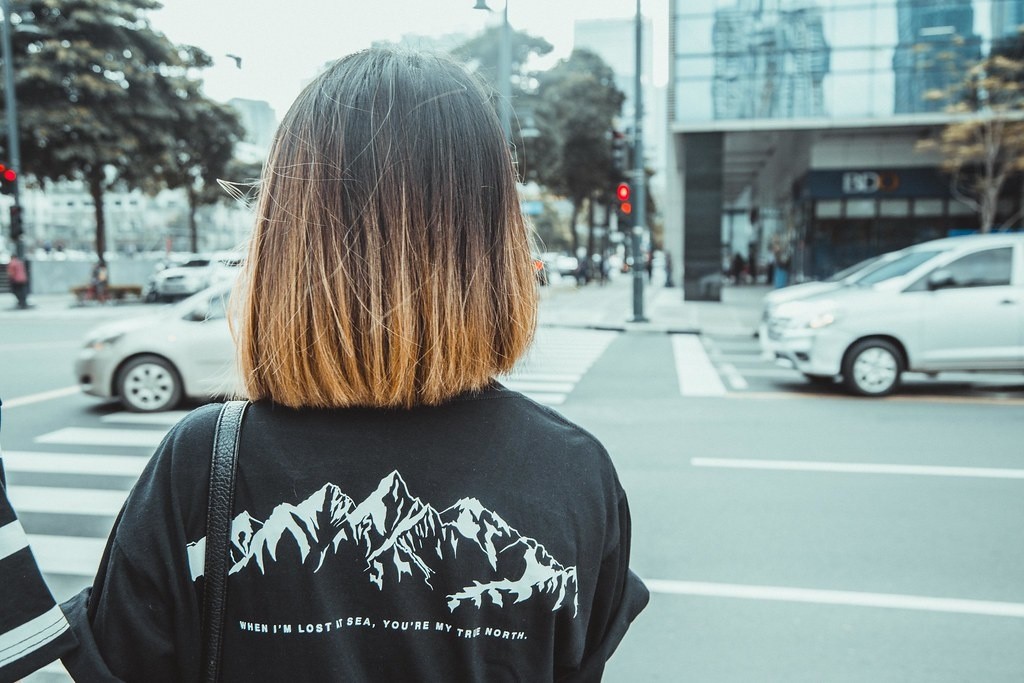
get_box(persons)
[7,255,29,308]
[733,237,791,289]
[59,46,650,683]
[93,255,109,303]
[0,399,80,683]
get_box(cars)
[754,230,1024,398]
[154,242,256,298]
[73,271,248,415]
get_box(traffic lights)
[9,204,25,238]
[0,166,17,196]
[616,182,630,208]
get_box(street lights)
[471,0,515,148]
